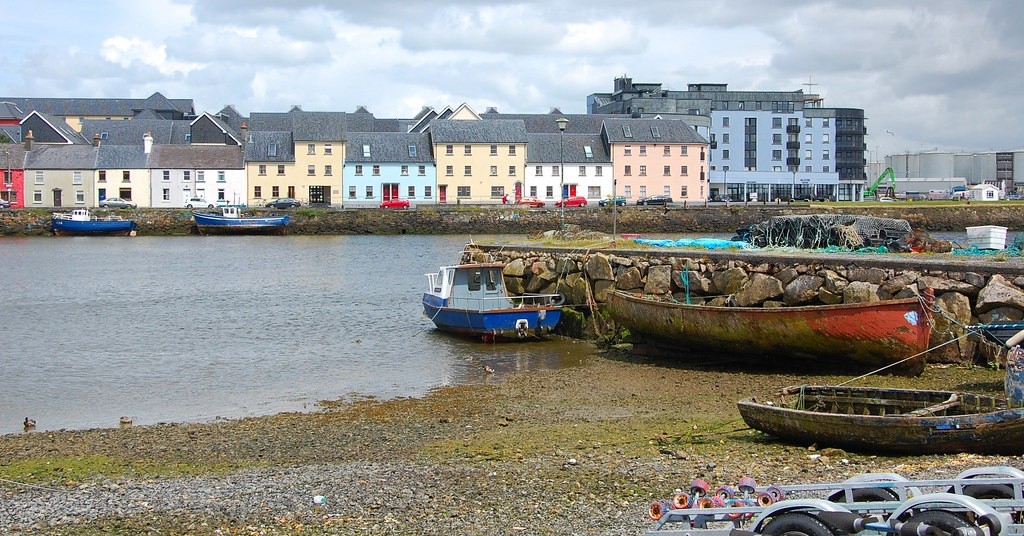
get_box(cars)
[555,196,587,207]
[516,198,545,208]
[266,198,302,209]
[184,197,216,209]
[99,198,137,209]
[880,197,897,202]
[599,196,626,206]
[636,195,673,206]
[710,194,732,202]
[0,199,9,208]
[380,198,410,209]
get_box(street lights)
[555,117,570,233]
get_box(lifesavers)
[548,291,566,306]
[463,252,471,262]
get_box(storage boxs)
[964,225,1008,251]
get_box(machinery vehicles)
[863,167,895,198]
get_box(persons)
[502,194,510,204]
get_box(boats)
[736,383,1024,456]
[604,286,934,376]
[51,208,136,236]
[527,224,608,240]
[191,207,291,236]
[422,262,562,342]
